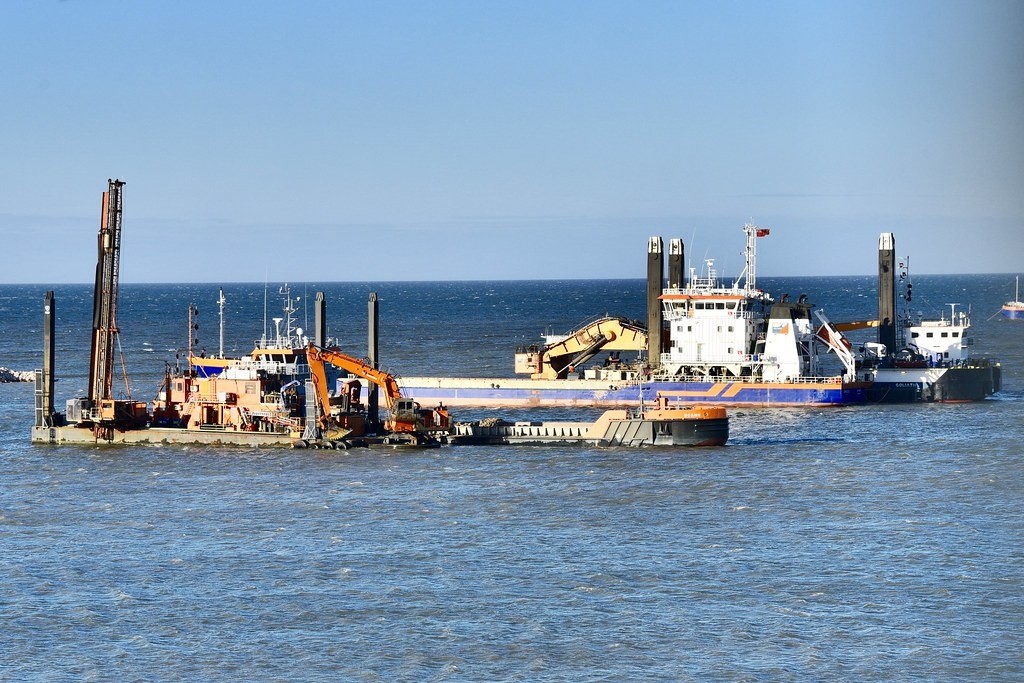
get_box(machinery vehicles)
[303,341,449,441]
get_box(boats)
[840,231,1003,405]
[593,215,887,409]
[1001,274,1024,319]
